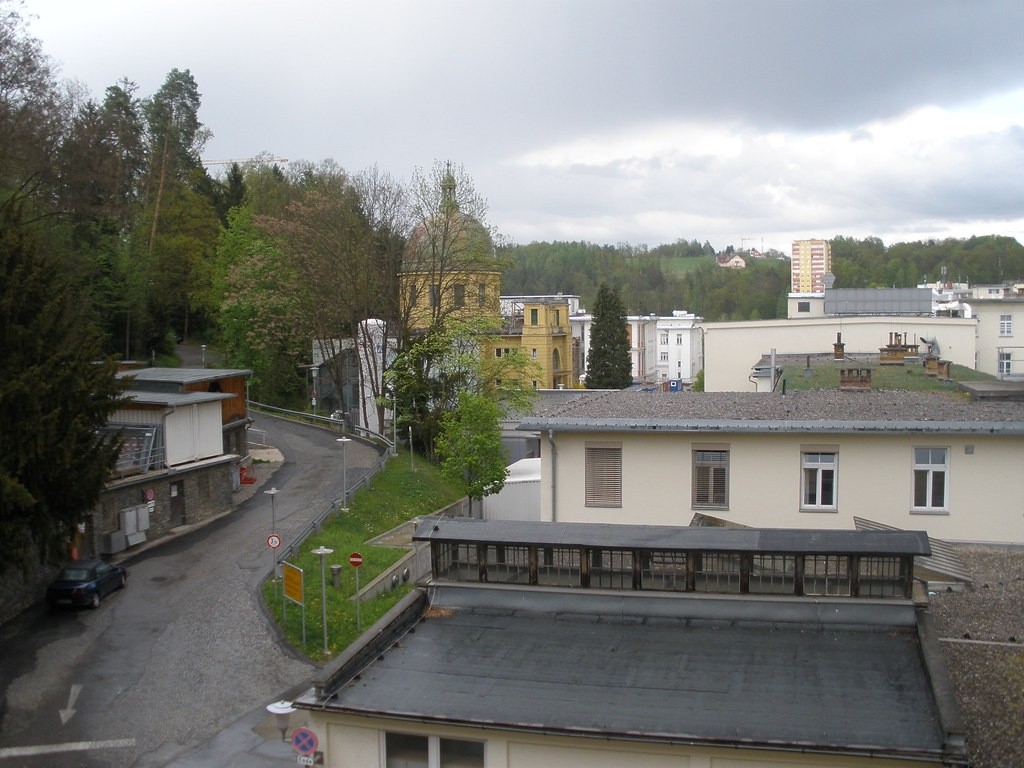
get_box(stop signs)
[349,552,363,567]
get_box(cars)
[46,558,128,609]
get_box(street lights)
[200,344,207,365]
[336,436,351,516]
[311,366,322,421]
[264,487,283,604]
[391,396,399,457]
[311,545,334,650]
